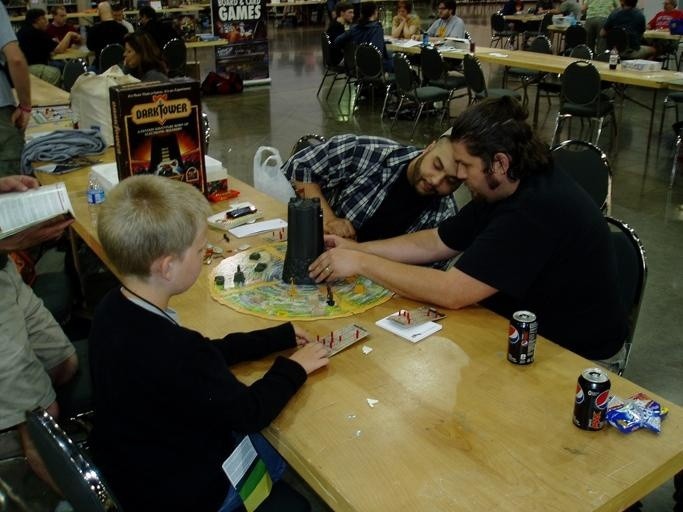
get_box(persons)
[0,174,80,500]
[1,1,189,173]
[325,1,466,102]
[308,95,630,360]
[282,126,459,271]
[87,174,330,511]
[497,1,682,61]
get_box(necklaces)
[120,283,178,325]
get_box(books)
[0,181,77,241]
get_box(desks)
[27,143,682,512]
[9,74,73,107]
[50,35,229,87]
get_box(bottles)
[609,46,618,70]
[470,42,475,52]
[423,32,428,45]
[87,175,106,228]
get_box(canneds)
[506,311,537,364]
[571,368,611,432]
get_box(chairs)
[663,121,682,224]
[59,54,88,93]
[553,136,613,215]
[600,210,648,373]
[96,41,124,73]
[160,36,186,74]
[20,399,123,512]
